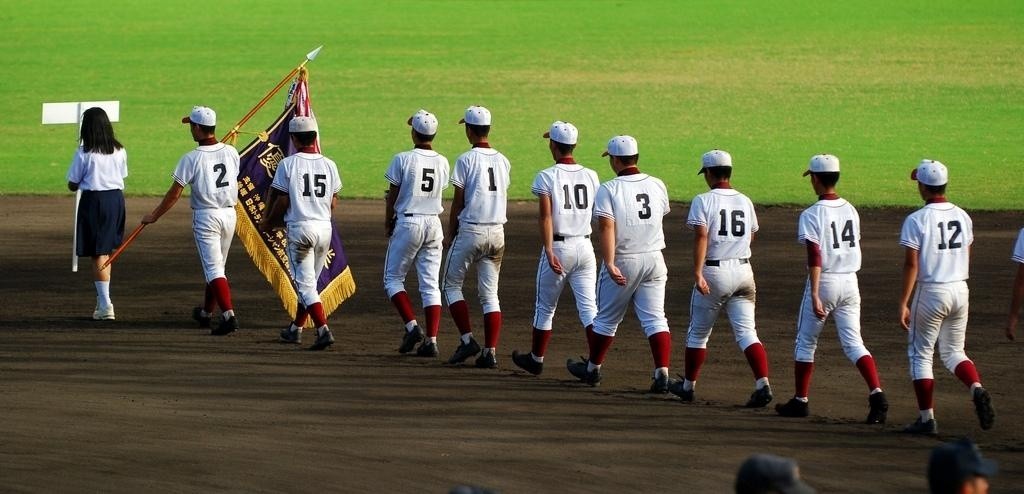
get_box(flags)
[223,70,357,329]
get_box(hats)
[929,440,997,476]
[734,454,816,494]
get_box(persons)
[664,148,775,409]
[731,451,815,491]
[895,159,997,439]
[376,107,451,360]
[515,114,602,377]
[776,155,893,427]
[924,434,1000,494]
[562,133,674,397]
[256,111,339,350]
[439,102,511,369]
[65,104,129,320]
[139,102,242,336]
[1005,224,1024,342]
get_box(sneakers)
[93,304,115,320]
